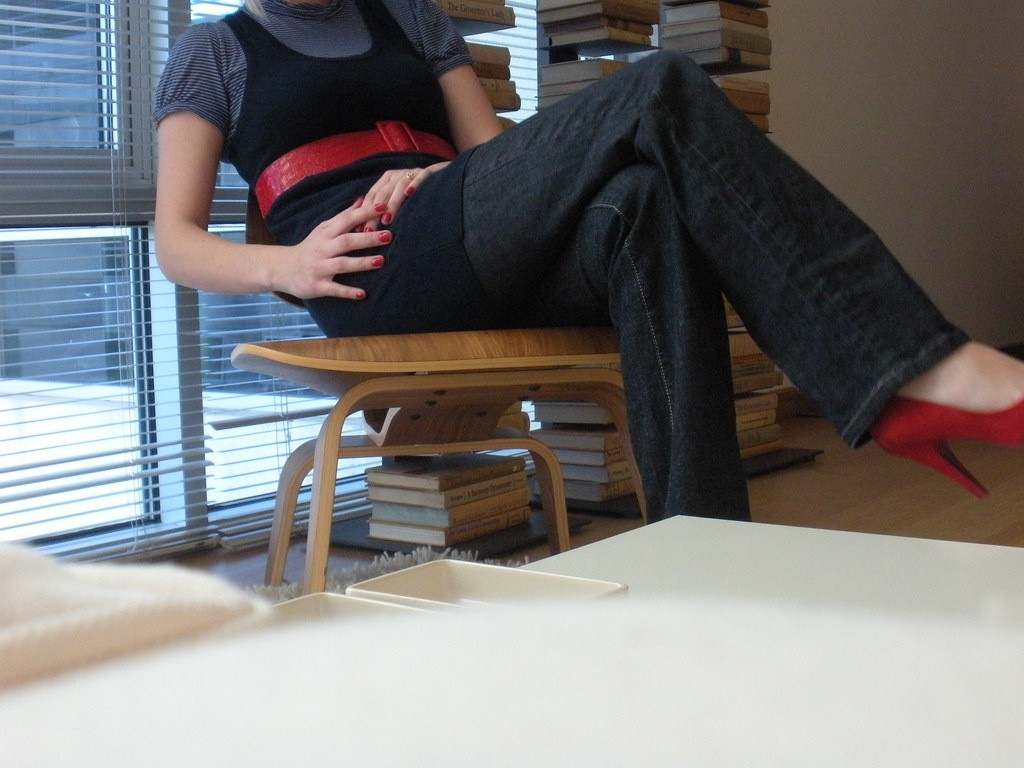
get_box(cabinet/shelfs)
[658,0,822,476]
[524,0,642,518]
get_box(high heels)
[868,394,1023,497]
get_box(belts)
[254,120,457,218]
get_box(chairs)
[230,180,649,597]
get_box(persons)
[152,0,1024,528]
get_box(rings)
[406,172,414,179]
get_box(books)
[529,399,636,503]
[434,0,772,135]
[364,397,530,548]
[719,292,784,461]
